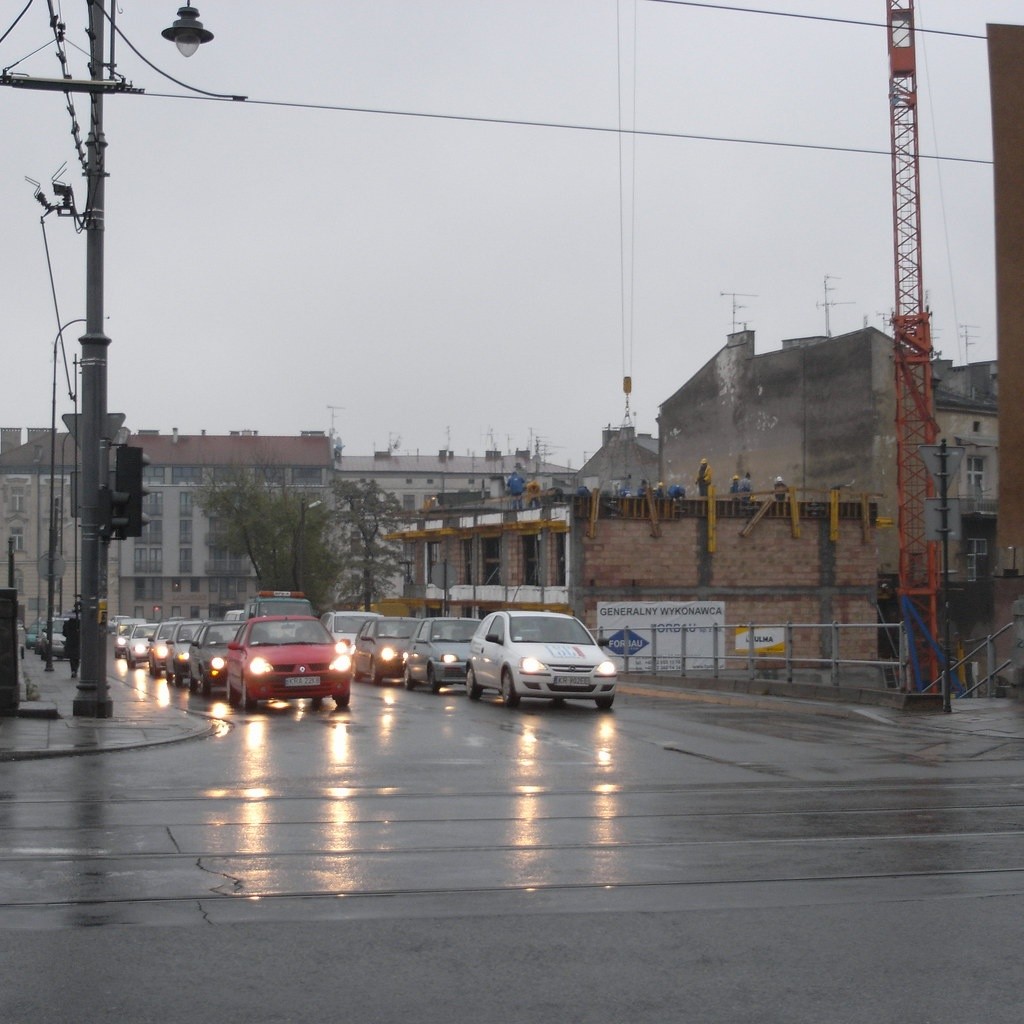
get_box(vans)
[243,591,315,620]
[222,610,245,623]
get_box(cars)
[463,610,619,709]
[146,620,181,678]
[188,620,244,698]
[34,634,42,655]
[403,616,482,693]
[225,613,352,710]
[125,623,159,669]
[25,623,40,650]
[114,619,145,659]
[352,616,421,684]
[111,615,130,622]
[165,619,209,689]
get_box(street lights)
[69,0,213,719]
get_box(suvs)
[321,608,385,657]
[40,617,69,658]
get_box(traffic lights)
[114,440,150,539]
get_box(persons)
[527,496,542,509]
[737,472,755,516]
[576,486,592,495]
[695,458,713,496]
[507,471,525,509]
[775,476,789,501]
[729,475,741,517]
[63,618,81,677]
[622,479,686,499]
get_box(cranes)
[883,1,950,698]
